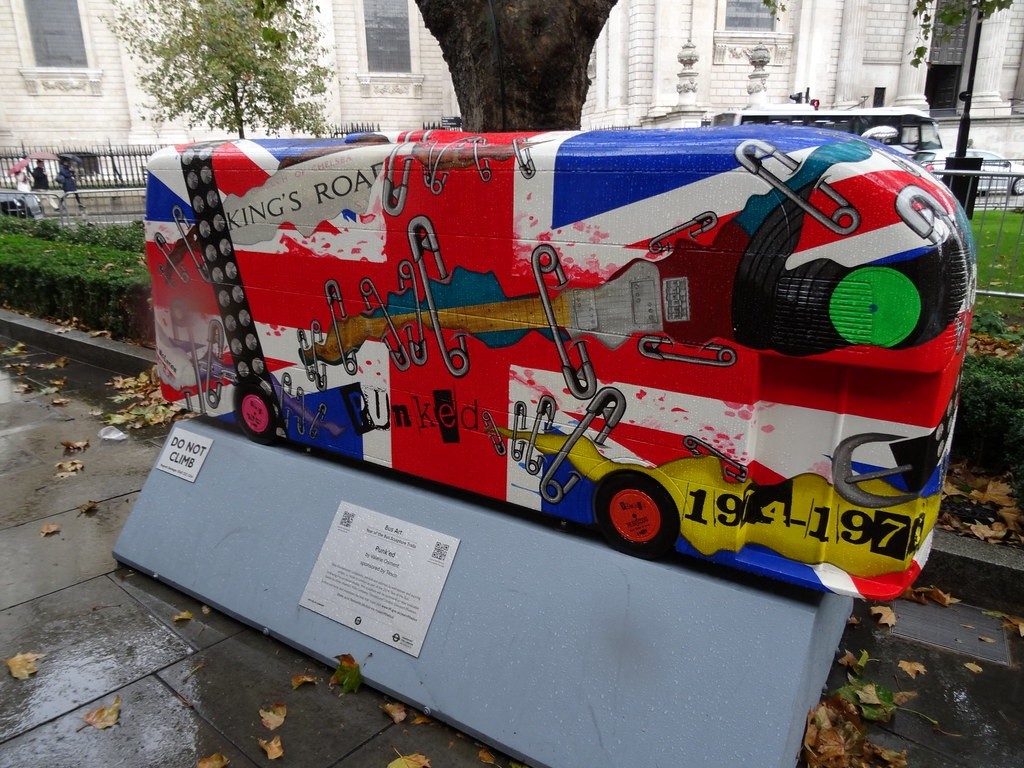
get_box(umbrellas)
[25,150,60,161]
[58,151,82,165]
[7,160,29,181]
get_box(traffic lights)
[789,92,803,103]
[810,99,820,108]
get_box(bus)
[731,104,943,178]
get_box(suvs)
[909,146,1024,194]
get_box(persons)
[966,137,976,150]
[14,167,42,216]
[55,158,86,211]
[27,158,59,211]
